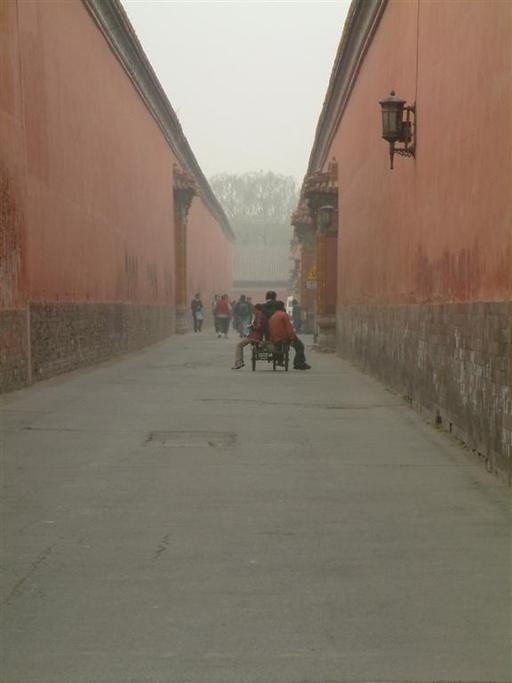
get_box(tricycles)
[251,333,290,372]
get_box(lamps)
[379,91,416,170]
[320,203,338,238]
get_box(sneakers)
[294,363,311,369]
[232,362,245,369]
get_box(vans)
[285,294,294,316]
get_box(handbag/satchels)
[195,312,204,320]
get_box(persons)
[191,292,232,339]
[232,291,311,370]
